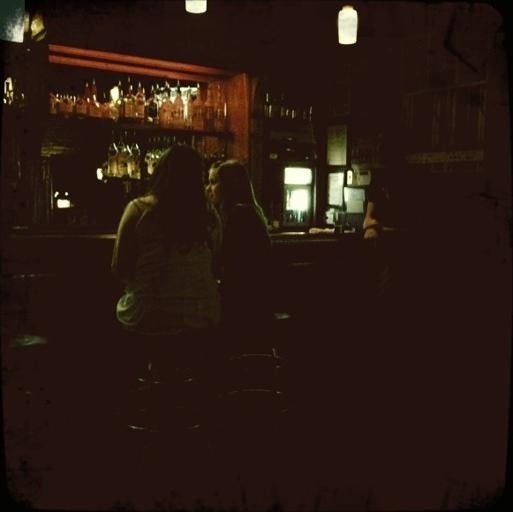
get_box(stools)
[111,307,292,439]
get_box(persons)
[110,146,272,335]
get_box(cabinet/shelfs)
[42,46,233,227]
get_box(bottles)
[107,130,173,182]
[79,76,229,131]
